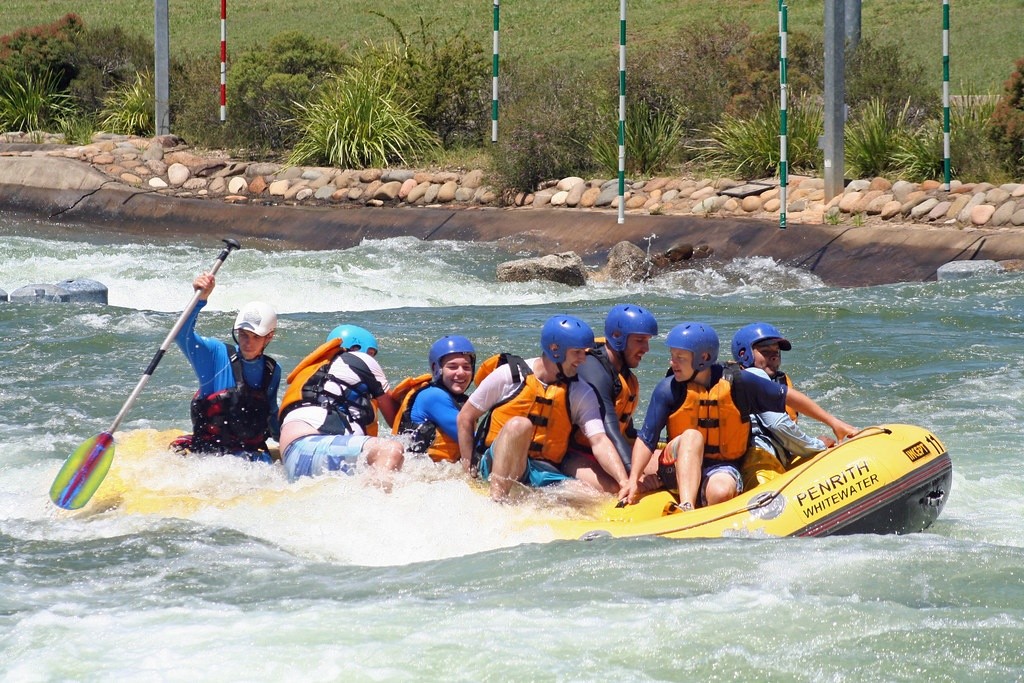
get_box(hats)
[757,338,791,351]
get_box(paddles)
[48,237,240,511]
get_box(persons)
[456,304,861,517]
[174,272,280,463]
[391,335,478,463]
[278,324,403,480]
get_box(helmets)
[325,324,378,356]
[605,304,658,351]
[664,322,719,371]
[234,301,277,337]
[428,335,476,383]
[731,323,780,368]
[541,315,594,363]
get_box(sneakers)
[661,501,691,517]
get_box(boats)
[88,417,954,555]
